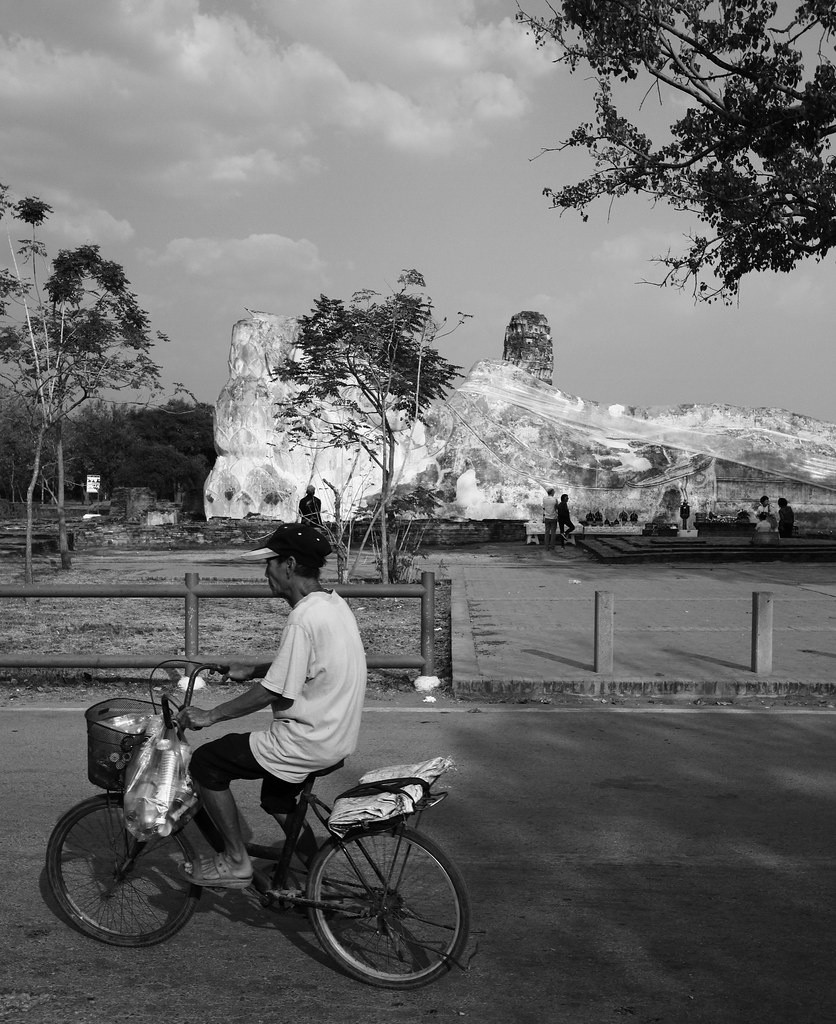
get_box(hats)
[241,523,332,568]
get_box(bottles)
[91,714,200,838]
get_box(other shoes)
[561,546,566,549]
[561,532,567,541]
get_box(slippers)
[179,853,254,889]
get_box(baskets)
[84,697,173,793]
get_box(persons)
[175,521,368,890]
[543,488,576,551]
[755,495,795,539]
[680,499,689,530]
[298,484,322,523]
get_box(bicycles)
[45,659,490,992]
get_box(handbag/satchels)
[123,715,198,843]
[767,514,778,529]
